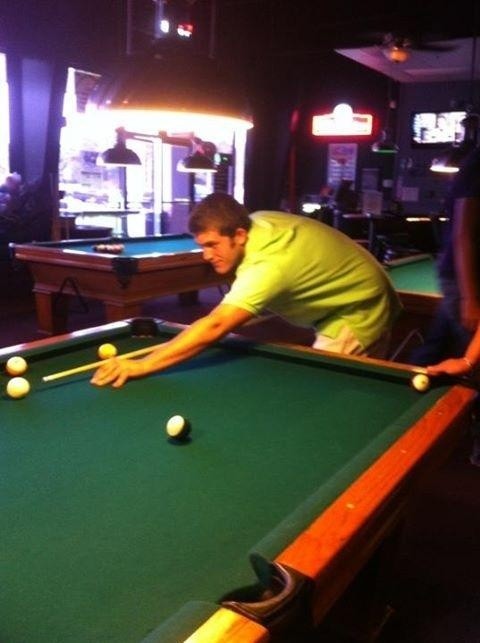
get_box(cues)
[42,313,277,383]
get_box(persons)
[425,321,480,379]
[317,177,406,221]
[415,125,480,368]
[419,114,455,143]
[89,191,404,389]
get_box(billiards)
[7,376,30,399]
[167,415,191,439]
[93,244,126,252]
[7,356,27,377]
[413,374,429,391]
[98,344,117,360]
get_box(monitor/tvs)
[413,109,468,145]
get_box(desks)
[8,233,235,335]
[379,250,449,336]
[1,314,480,641]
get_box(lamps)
[370,127,401,154]
[426,146,461,174]
[91,0,255,129]
[95,127,222,175]
[378,38,409,62]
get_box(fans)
[332,1,461,66]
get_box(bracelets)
[463,357,474,377]
